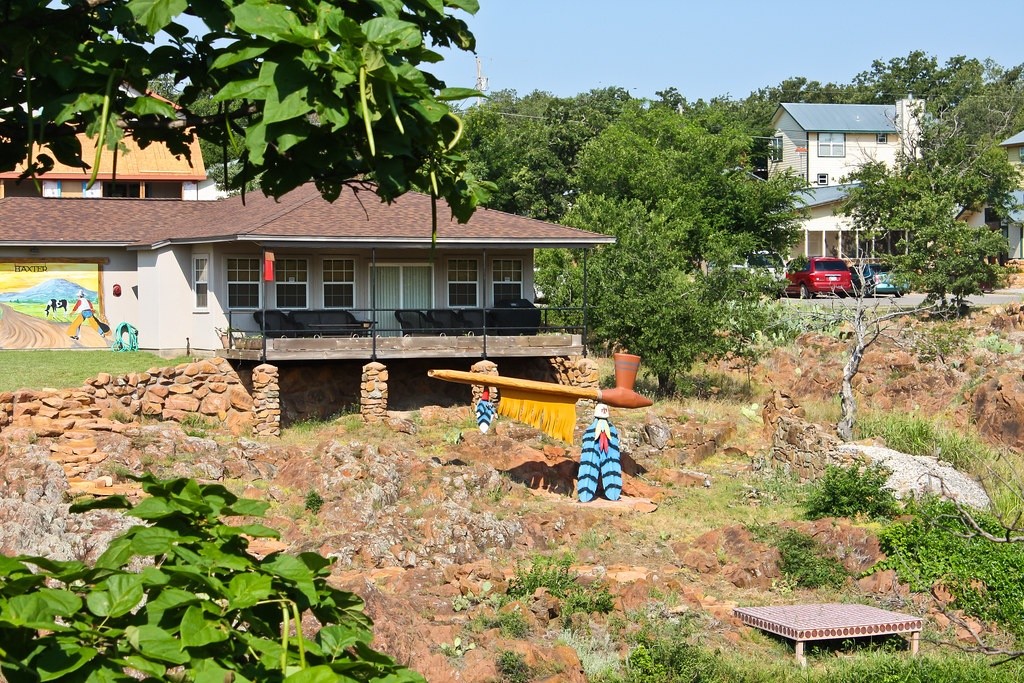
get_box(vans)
[712,250,790,299]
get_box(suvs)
[777,255,853,301]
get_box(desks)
[307,324,363,337]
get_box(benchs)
[288,310,379,338]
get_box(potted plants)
[529,328,572,346]
[220,328,265,350]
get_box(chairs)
[393,298,542,336]
[252,310,303,338]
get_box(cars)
[847,262,909,300]
